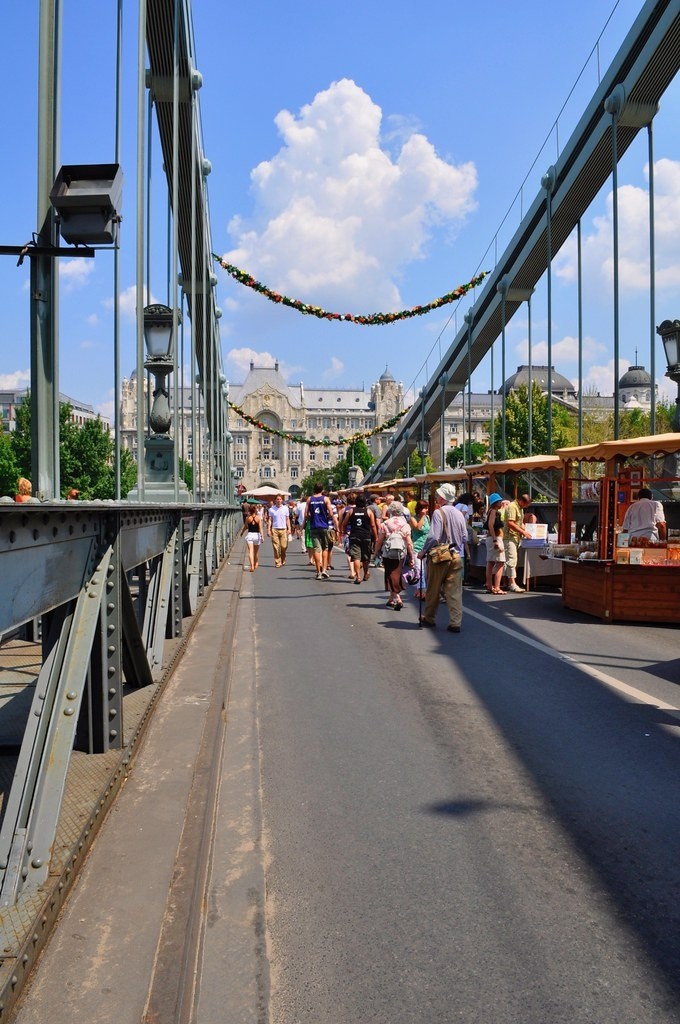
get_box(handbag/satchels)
[482,510,500,533]
[429,542,453,564]
[399,576,407,590]
[406,566,419,585]
[466,526,478,545]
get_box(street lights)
[417,430,432,474]
[126,305,191,502]
[648,319,680,501]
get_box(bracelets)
[492,540,497,542]
[468,555,470,557]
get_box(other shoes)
[419,615,436,626]
[510,585,526,593]
[422,593,426,601]
[413,591,420,598]
[439,598,446,603]
[249,544,376,583]
[448,626,460,632]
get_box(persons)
[241,483,539,612]
[622,489,666,545]
[267,494,292,568]
[419,483,468,633]
[373,501,415,610]
[67,487,81,499]
[15,477,33,502]
[240,505,264,572]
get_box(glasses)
[474,496,477,498]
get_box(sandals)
[386,599,394,607]
[394,602,403,611]
[486,587,494,593]
[493,589,507,594]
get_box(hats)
[488,493,503,505]
[436,483,457,502]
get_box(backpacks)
[381,520,407,559]
